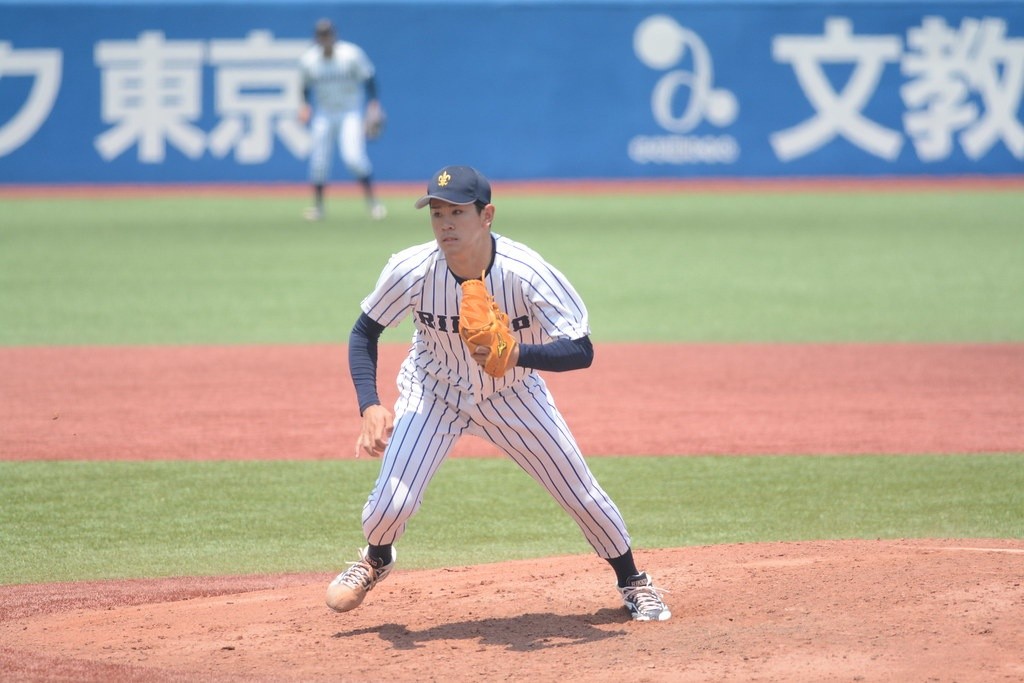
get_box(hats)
[414,164,491,210]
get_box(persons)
[326,168,672,622]
[294,19,386,222]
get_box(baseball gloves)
[458,269,518,379]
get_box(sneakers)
[325,545,396,613]
[615,572,672,621]
